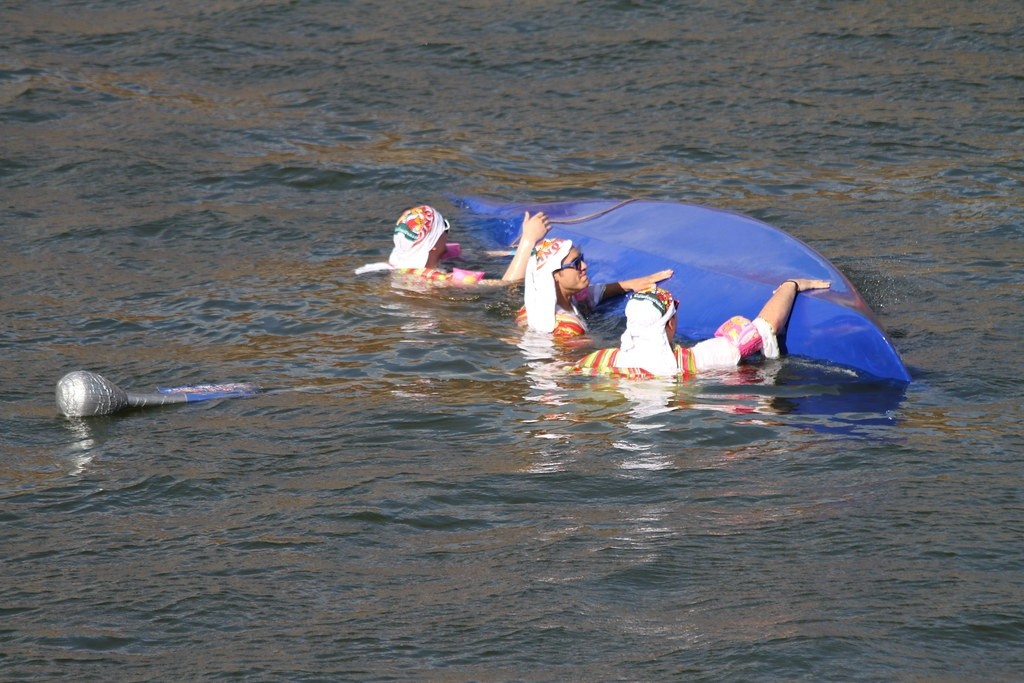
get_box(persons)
[579,278,831,374]
[389,206,552,280]
[516,238,673,334]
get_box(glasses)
[441,217,451,233]
[557,253,584,274]
[663,300,680,328]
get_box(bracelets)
[782,280,799,291]
